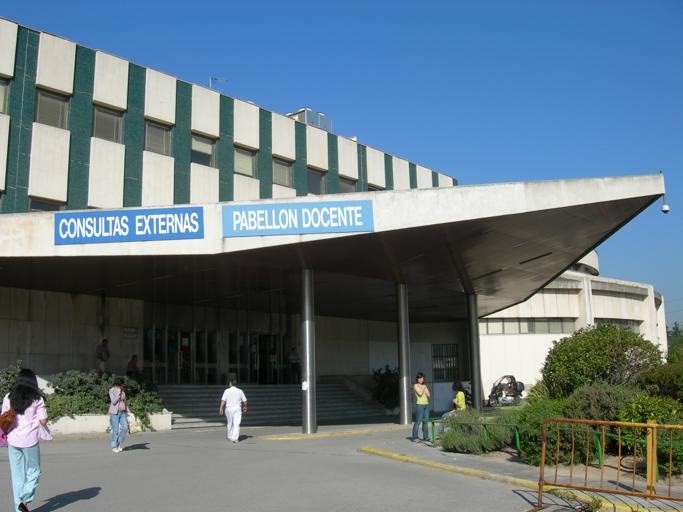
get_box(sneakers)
[424,437,431,441]
[412,438,420,442]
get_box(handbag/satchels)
[0,410,19,434]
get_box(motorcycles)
[488,374,524,407]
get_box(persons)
[412,372,430,442]
[220,380,247,443]
[126,355,138,377]
[438,382,466,435]
[1,369,53,512]
[96,339,110,373]
[289,346,301,384]
[109,377,131,453]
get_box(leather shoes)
[113,448,119,453]
[118,445,123,451]
[18,503,31,512]
